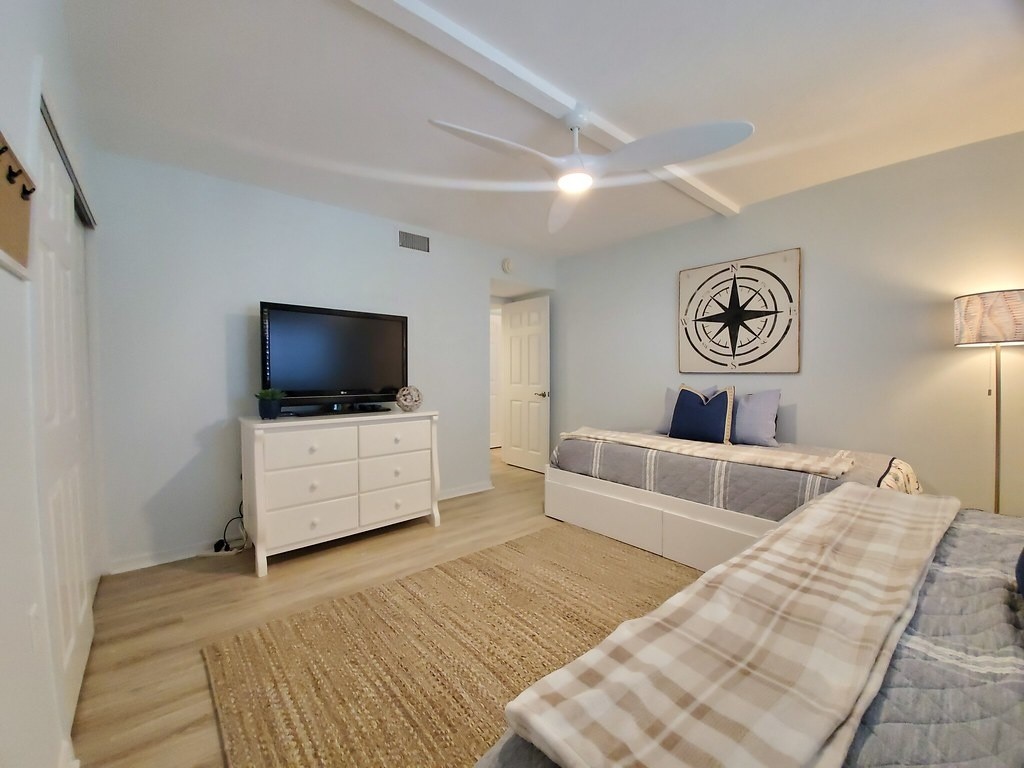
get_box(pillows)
[667,382,735,447]
[655,385,719,434]
[728,389,781,447]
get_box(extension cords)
[198,549,238,557]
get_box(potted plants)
[255,388,286,420]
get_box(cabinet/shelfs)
[238,409,440,577]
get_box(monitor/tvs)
[259,301,408,417]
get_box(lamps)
[953,289,1024,515]
[555,171,594,196]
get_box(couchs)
[472,481,1024,768]
[545,427,923,573]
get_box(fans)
[428,111,754,235]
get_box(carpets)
[201,521,706,768]
